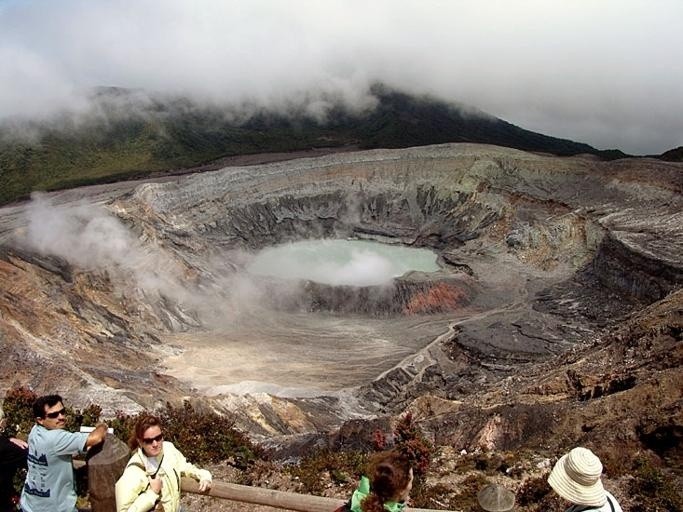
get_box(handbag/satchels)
[148,500,165,512]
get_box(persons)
[115,415,212,511]
[20,392,108,512]
[0,406,28,512]
[332,448,414,511]
[547,447,624,512]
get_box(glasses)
[45,407,65,418]
[143,433,162,443]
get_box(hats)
[547,447,607,506]
